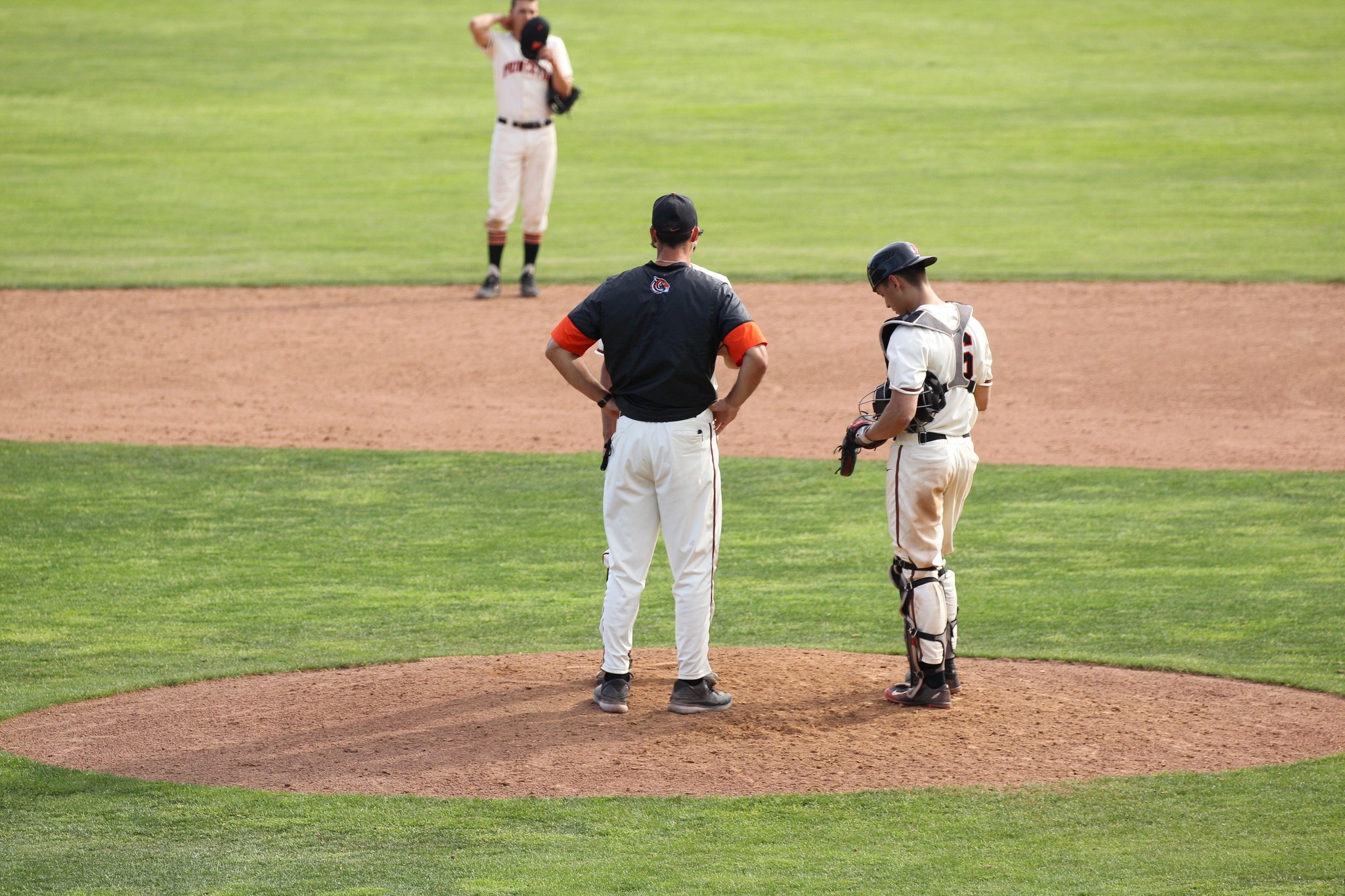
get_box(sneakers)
[517,272,539,299]
[592,670,637,713]
[905,671,967,696]
[666,672,733,714]
[884,682,952,709]
[476,273,504,297]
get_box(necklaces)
[654,259,687,263]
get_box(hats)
[519,16,552,61]
[867,241,938,291]
[652,191,699,233]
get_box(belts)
[495,117,554,129]
[893,432,971,446]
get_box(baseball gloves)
[546,75,579,115]
[839,415,890,477]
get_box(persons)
[834,242,994,709]
[543,192,768,714]
[469,0,581,298]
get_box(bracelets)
[597,393,612,409]
